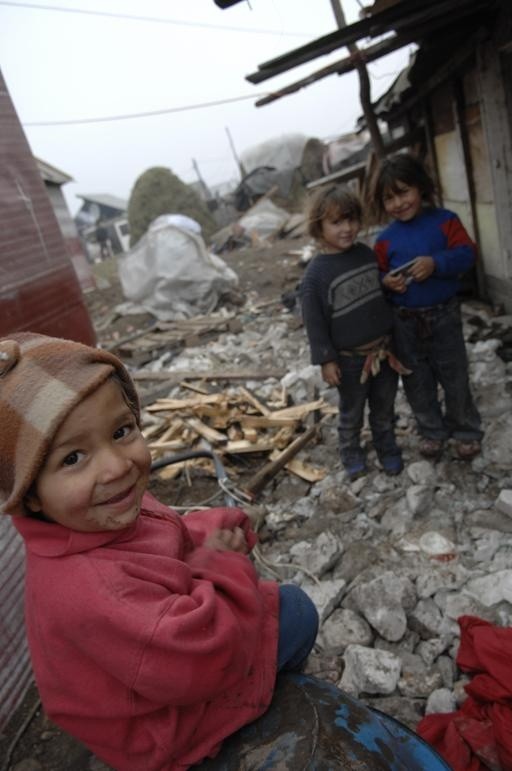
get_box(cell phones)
[388,261,416,279]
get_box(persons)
[300,185,406,482]
[0,328,320,771]
[371,155,488,461]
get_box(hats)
[0,330,141,515]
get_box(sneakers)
[416,435,442,458]
[381,453,403,474]
[346,459,367,480]
[454,436,482,459]
